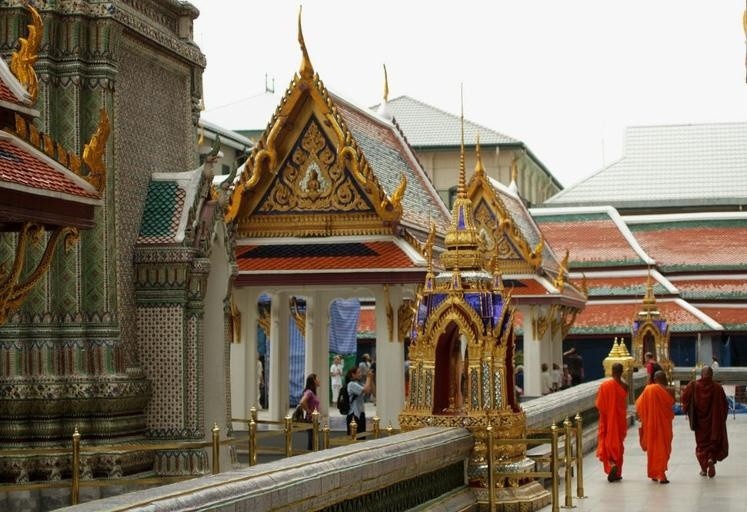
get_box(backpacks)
[652,363,662,376]
[337,380,358,415]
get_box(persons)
[634,370,676,484]
[644,351,661,387]
[681,365,729,477]
[710,356,719,368]
[299,374,321,450]
[405,353,410,401]
[515,365,524,396]
[594,362,630,483]
[306,170,320,191]
[256,352,265,410]
[330,354,376,440]
[541,346,586,396]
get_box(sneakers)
[608,467,622,481]
[701,459,715,477]
[653,479,669,483]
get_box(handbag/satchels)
[293,405,307,422]
